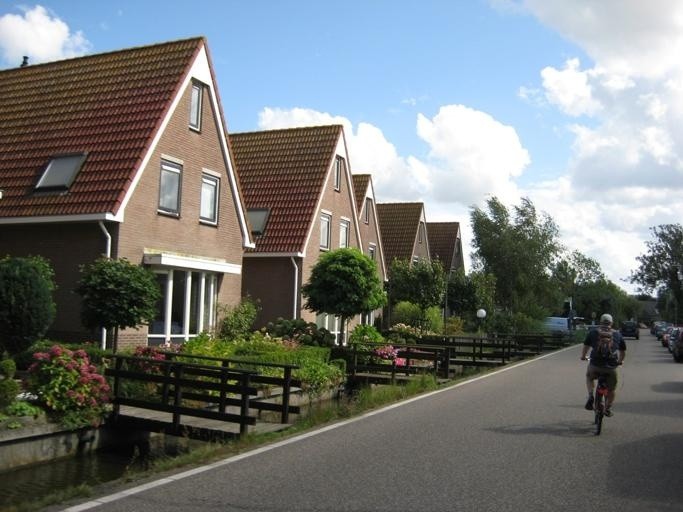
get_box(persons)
[581,312,628,418]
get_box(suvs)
[619,320,639,340]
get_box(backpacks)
[592,328,618,365]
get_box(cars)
[651,320,683,362]
[638,322,648,330]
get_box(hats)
[599,313,613,324]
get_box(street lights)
[476,308,487,359]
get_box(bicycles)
[579,356,623,436]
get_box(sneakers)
[604,409,613,417]
[584,397,593,410]
